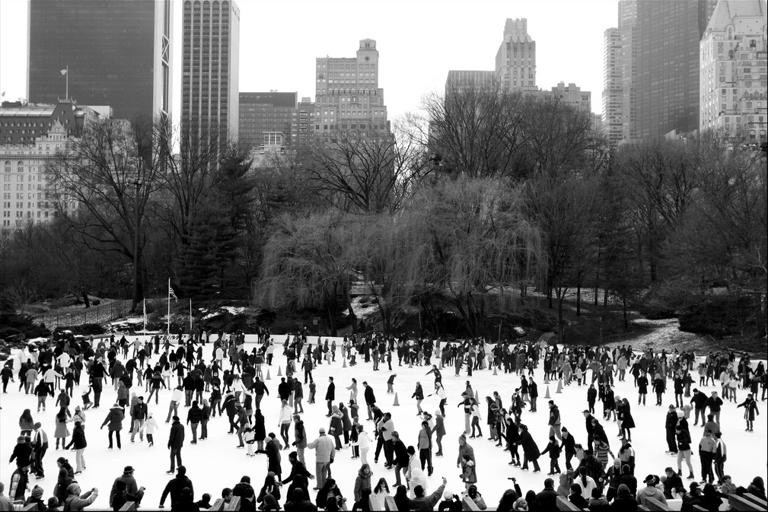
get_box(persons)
[2,326,768,511]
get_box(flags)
[169,286,177,298]
